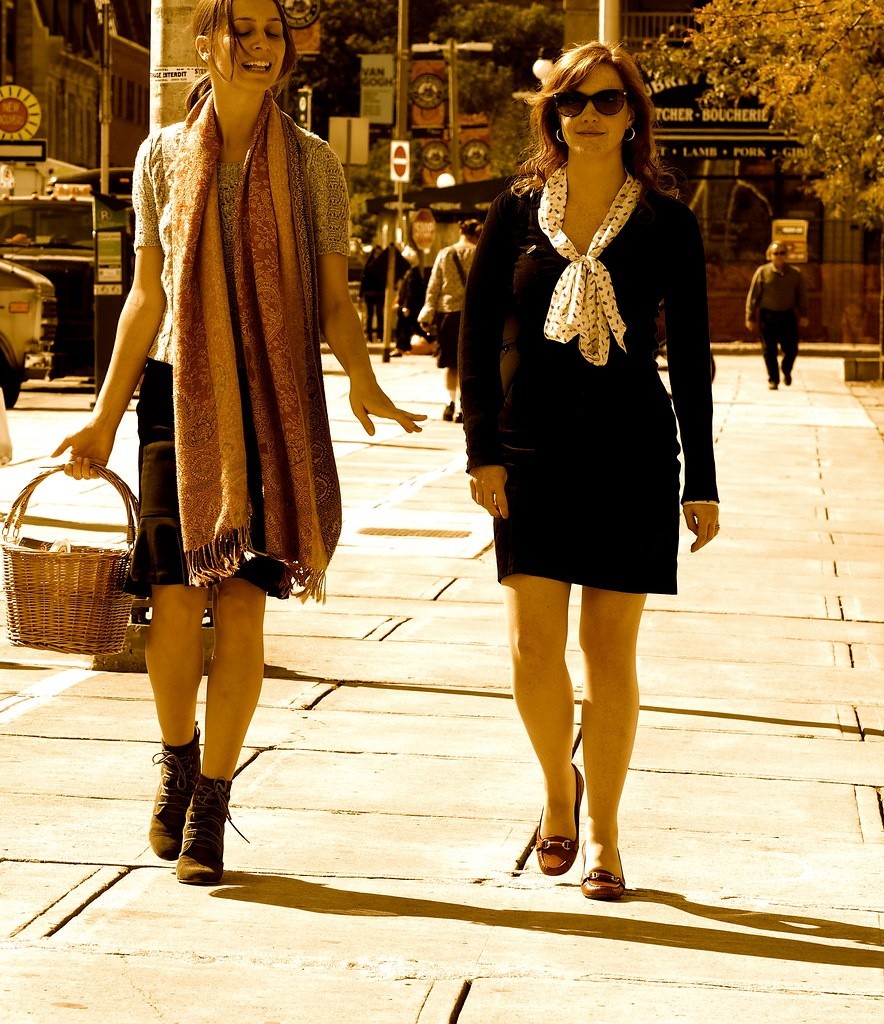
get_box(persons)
[458,45,720,901]
[742,242,808,390]
[50,0,428,886]
[359,218,482,424]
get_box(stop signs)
[390,138,411,185]
[410,207,436,251]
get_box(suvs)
[0,256,58,407]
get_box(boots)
[149,721,200,861]
[176,774,250,884]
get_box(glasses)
[553,88,628,117]
[770,251,787,257]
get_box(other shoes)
[769,380,777,390]
[537,762,584,876]
[444,402,454,421]
[581,839,624,899]
[456,411,463,424]
[784,373,792,386]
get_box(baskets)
[1,459,140,655]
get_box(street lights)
[411,37,494,183]
